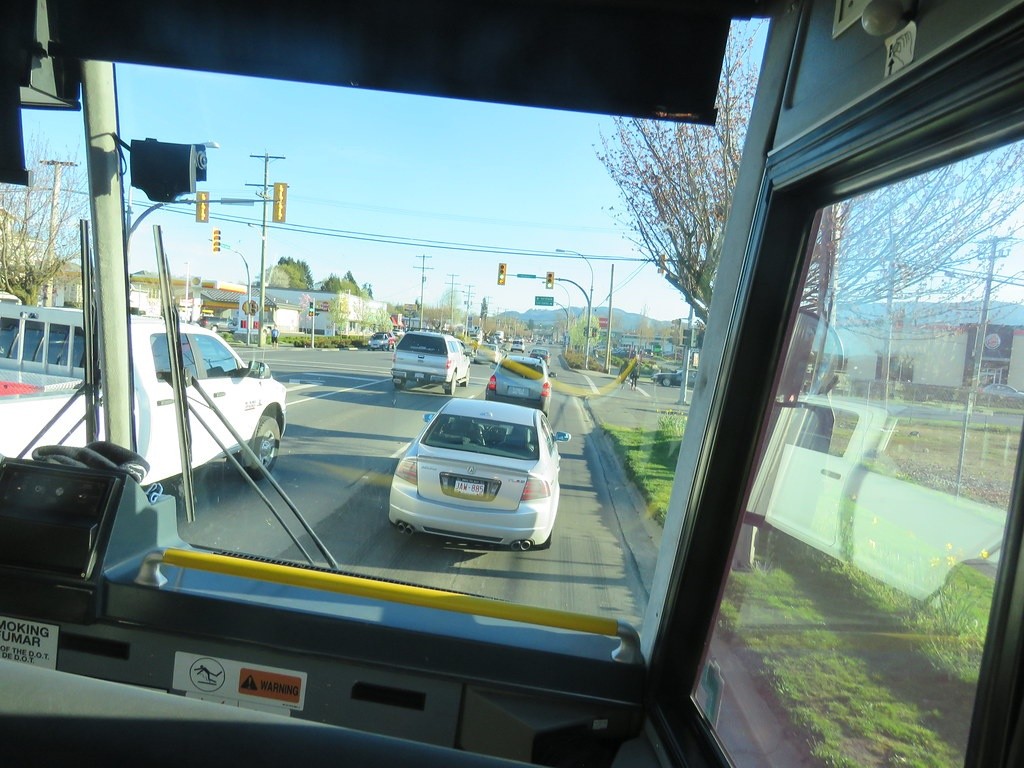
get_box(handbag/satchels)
[630,374,634,378]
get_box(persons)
[619,357,629,389]
[387,335,394,350]
[630,357,640,390]
[272,325,279,348]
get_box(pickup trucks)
[762,394,1013,617]
[0,305,289,524]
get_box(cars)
[529,348,551,368]
[511,339,553,353]
[472,329,508,364]
[652,369,697,388]
[612,346,636,358]
[367,329,405,351]
[389,398,572,551]
[979,384,1024,402]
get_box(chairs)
[152,336,170,370]
[447,418,477,443]
[506,427,534,451]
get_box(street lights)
[556,248,594,369]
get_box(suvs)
[390,331,471,395]
[485,354,556,416]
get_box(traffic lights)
[545,272,554,289]
[670,319,682,345]
[309,302,313,315]
[656,255,664,274]
[498,263,506,285]
[213,228,222,254]
[683,330,692,346]
